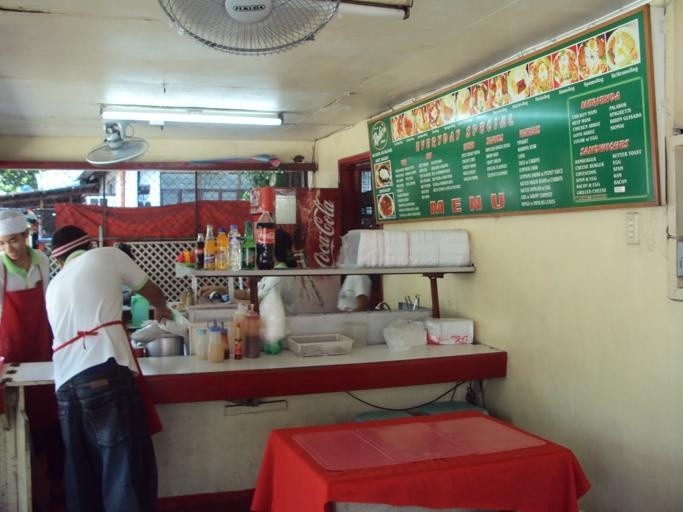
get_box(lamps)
[276,1,414,20]
[99,104,284,126]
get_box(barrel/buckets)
[143,332,184,357]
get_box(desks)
[252,412,591,511]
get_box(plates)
[390,26,639,143]
[377,166,395,217]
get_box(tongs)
[404,295,421,310]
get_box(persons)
[109,242,136,306]
[196,229,301,318]
[42,222,176,512]
[336,274,373,313]
[0,202,60,512]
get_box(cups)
[130,294,150,326]
[341,321,368,348]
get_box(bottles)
[196,210,278,270]
[195,303,259,362]
[178,251,195,264]
[289,245,326,309]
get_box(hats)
[0,209,29,237]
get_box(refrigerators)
[250,187,342,313]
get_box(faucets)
[374,300,390,313]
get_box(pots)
[146,336,186,357]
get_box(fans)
[85,122,149,167]
[158,0,340,56]
